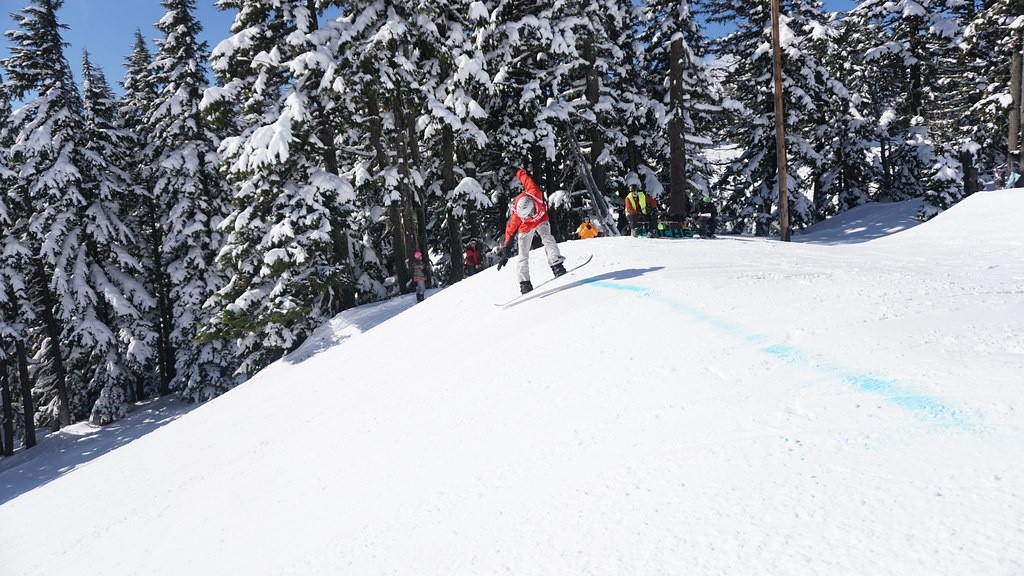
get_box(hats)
[415,252,422,258]
[470,239,476,243]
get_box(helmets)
[703,196,711,202]
[517,196,533,217]
[629,184,638,192]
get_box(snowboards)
[495,253,597,309]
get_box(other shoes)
[711,234,717,238]
[520,281,533,294]
[701,234,706,238]
[631,228,637,236]
[416,293,424,301]
[648,231,654,235]
[551,262,567,276]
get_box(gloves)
[506,165,519,177]
[497,257,508,271]
[587,225,591,230]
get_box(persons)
[413,251,427,303]
[690,194,718,239]
[577,215,598,240]
[465,239,480,276]
[496,164,567,296]
[624,183,660,238]
